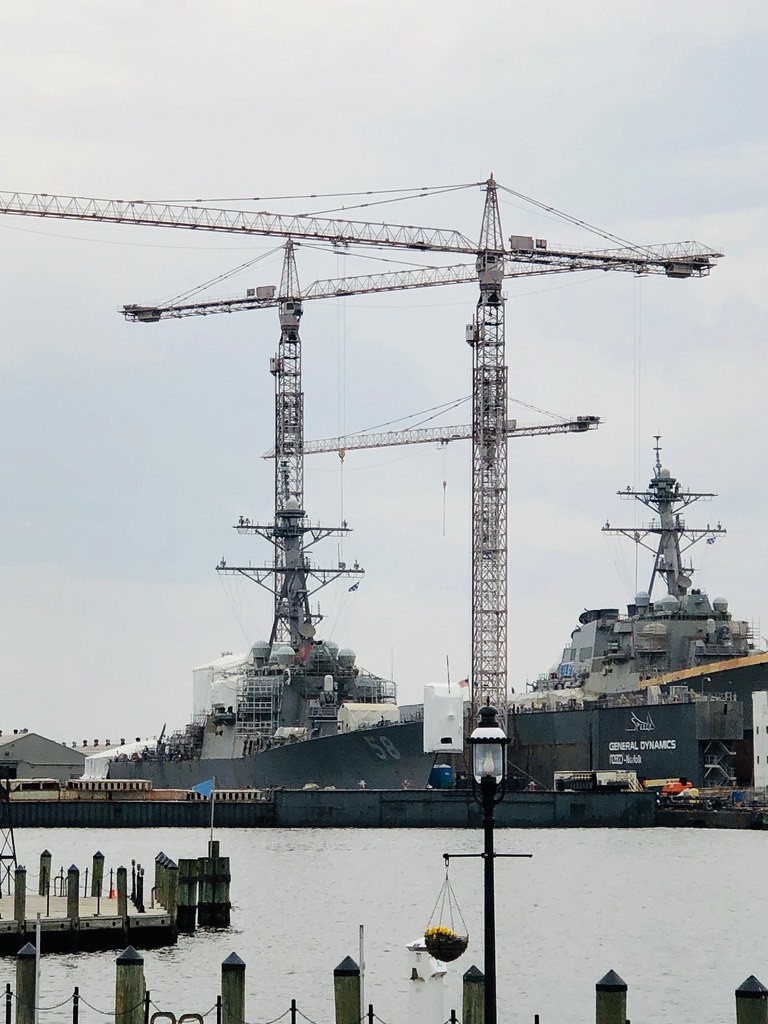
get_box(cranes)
[0,172,726,735]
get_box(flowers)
[424,926,457,942]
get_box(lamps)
[469,695,510,785]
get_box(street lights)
[464,697,513,1024]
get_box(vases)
[425,937,469,963]
